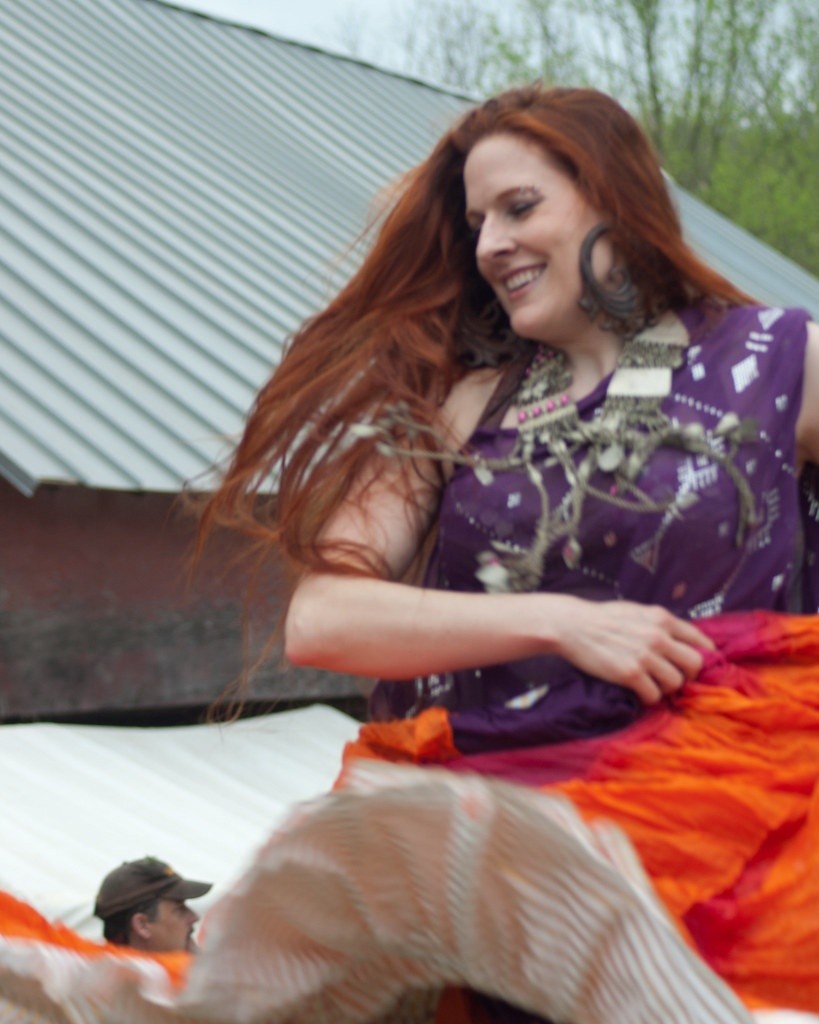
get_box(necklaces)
[373,318,758,593]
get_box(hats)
[94,856,212,920]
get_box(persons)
[94,858,212,952]
[0,88,819,1024]
